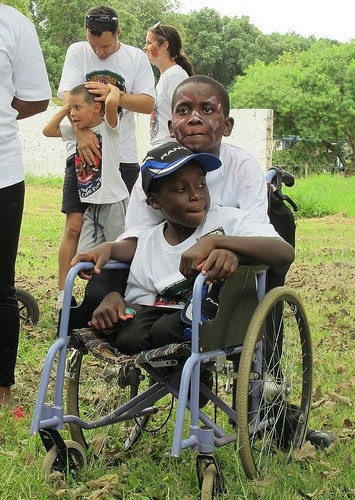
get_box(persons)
[0,2,53,407]
[42,7,330,448]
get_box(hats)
[140,141,222,206]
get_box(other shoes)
[274,402,332,450]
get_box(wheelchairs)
[26,163,314,500]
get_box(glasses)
[153,21,169,40]
